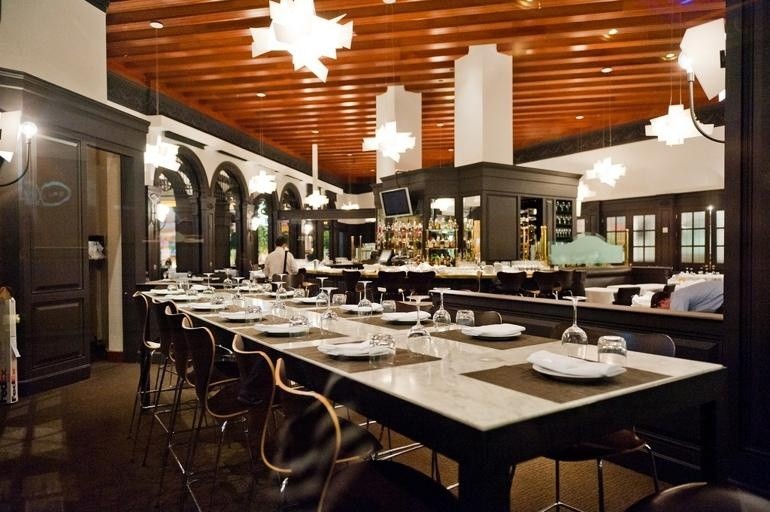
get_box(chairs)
[377,310,503,489]
[274,356,466,512]
[377,270,406,303]
[127,290,198,464]
[233,335,385,511]
[142,290,222,467]
[528,271,559,300]
[540,324,674,511]
[155,305,250,507]
[408,271,436,303]
[625,481,768,512]
[462,271,483,293]
[342,270,375,305]
[555,268,578,300]
[495,271,527,299]
[182,318,252,512]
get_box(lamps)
[144,133,183,175]
[302,189,331,210]
[577,179,596,202]
[680,54,725,145]
[341,201,360,210]
[587,157,626,188]
[0,121,37,188]
[249,0,359,85]
[248,169,278,195]
[362,121,417,162]
[680,18,726,102]
[646,105,713,147]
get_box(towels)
[461,323,527,338]
[190,284,215,291]
[187,302,225,309]
[380,311,431,322]
[219,311,263,320]
[234,284,263,292]
[292,298,327,304]
[316,339,387,357]
[527,349,627,378]
[164,295,201,300]
[253,322,310,333]
[150,288,184,294]
[263,292,295,297]
[340,303,384,312]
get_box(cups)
[288,309,310,342]
[248,270,261,296]
[203,273,214,302]
[455,309,476,327]
[276,273,287,297]
[315,276,329,310]
[293,288,305,299]
[332,293,347,309]
[231,276,245,309]
[556,201,559,212]
[556,227,572,238]
[597,336,626,368]
[406,295,432,354]
[319,286,341,337]
[209,294,227,316]
[222,267,233,290]
[560,202,566,212]
[381,299,396,314]
[271,281,289,324]
[357,281,373,317]
[557,215,562,224]
[367,331,396,369]
[244,305,263,327]
[429,288,452,333]
[561,296,588,359]
[566,215,571,224]
[563,215,566,224]
[566,202,571,213]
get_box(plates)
[253,324,310,336]
[218,311,264,321]
[530,363,627,380]
[463,332,522,340]
[187,302,225,310]
[380,317,428,322]
[316,346,392,357]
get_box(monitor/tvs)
[378,186,414,218]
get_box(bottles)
[453,219,457,229]
[440,253,444,264]
[435,254,440,265]
[378,218,422,232]
[446,252,450,265]
[434,218,440,229]
[435,236,440,248]
[440,218,446,229]
[443,255,447,264]
[429,218,434,229]
[448,220,453,229]
[444,237,449,247]
[431,238,435,247]
[378,233,422,249]
[440,239,444,249]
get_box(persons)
[651,281,724,314]
[164,260,176,273]
[265,235,299,281]
